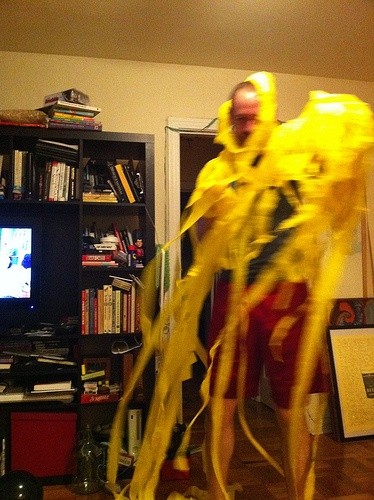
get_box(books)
[34,88,102,132]
[82,164,145,203]
[81,275,145,335]
[82,220,133,266]
[0,138,79,202]
[0,325,77,403]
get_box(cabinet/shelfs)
[0,123,156,483]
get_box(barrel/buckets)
[68,426,104,492]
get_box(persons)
[184,81,315,500]
[134,239,145,264]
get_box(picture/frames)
[328,325,374,442]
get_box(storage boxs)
[11,409,77,480]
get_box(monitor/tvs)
[0,216,43,314]
